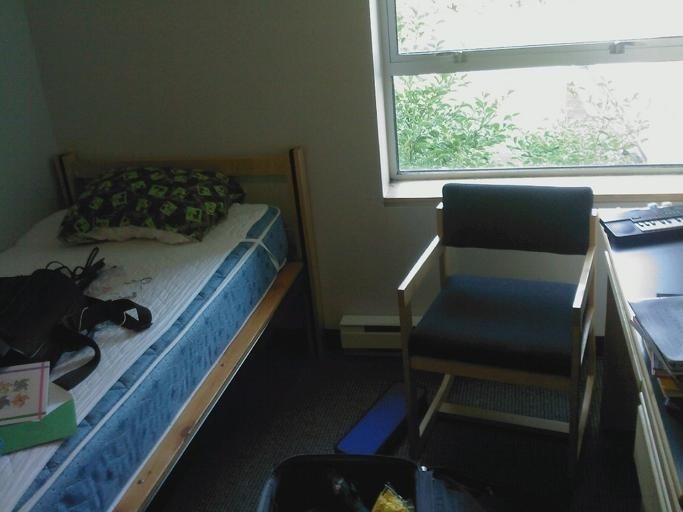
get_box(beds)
[0,145,324,510]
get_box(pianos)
[602,204,682,244]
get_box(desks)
[600,208,683,511]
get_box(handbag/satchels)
[0,268,88,368]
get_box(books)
[625,295,682,414]
[0,385,77,458]
[0,361,53,422]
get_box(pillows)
[57,166,246,245]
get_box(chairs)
[396,181,599,463]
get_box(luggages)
[255,452,502,512]
